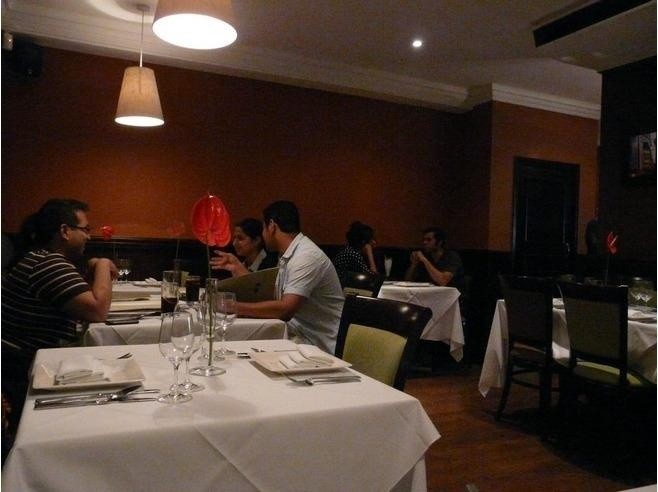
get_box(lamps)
[112,1,171,132]
[149,1,242,50]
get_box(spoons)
[286,375,362,386]
[33,384,142,406]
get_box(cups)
[186,275,200,302]
[161,282,178,312]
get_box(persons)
[232,217,276,273]
[332,219,381,299]
[1,196,121,417]
[208,199,345,356]
[4,213,93,283]
[404,226,470,315]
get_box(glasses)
[75,225,90,230]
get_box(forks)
[117,352,133,358]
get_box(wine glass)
[630,277,643,314]
[116,259,125,282]
[212,290,236,359]
[155,311,194,403]
[641,279,654,313]
[123,258,132,284]
[196,291,226,377]
[172,303,206,392]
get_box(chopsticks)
[35,387,160,404]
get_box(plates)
[247,350,353,374]
[32,357,145,390]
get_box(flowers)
[98,224,116,261]
[188,193,235,365]
